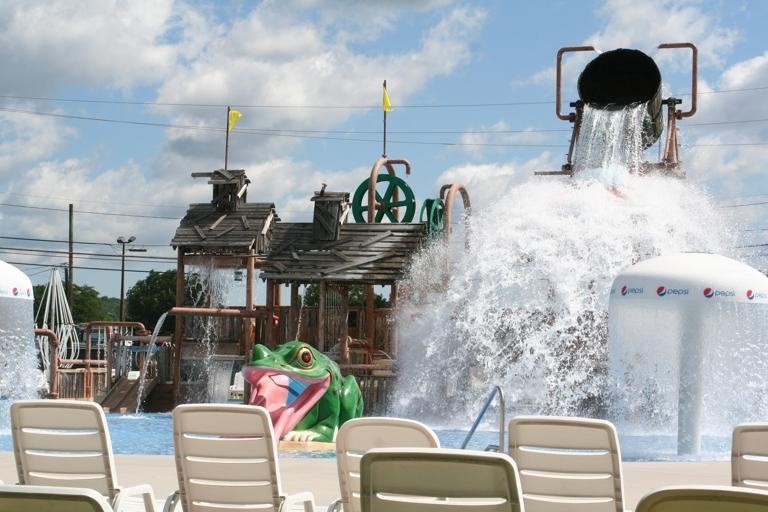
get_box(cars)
[82,328,105,344]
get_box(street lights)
[114,234,136,322]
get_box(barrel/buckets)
[578,48,663,151]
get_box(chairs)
[0,400,768,510]
